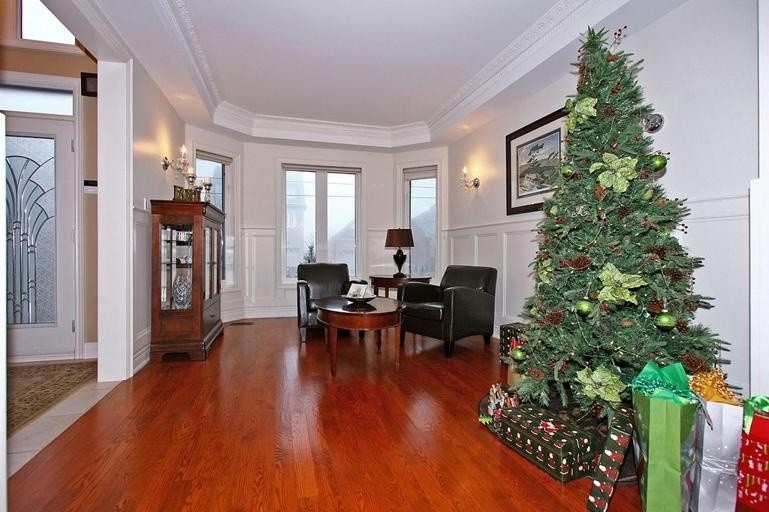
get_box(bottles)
[172,269,192,310]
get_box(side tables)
[368,274,432,302]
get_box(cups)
[173,185,201,201]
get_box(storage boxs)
[500,404,596,483]
[499,321,528,366]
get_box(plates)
[341,294,377,304]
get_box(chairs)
[401,265,497,358]
[297,263,368,343]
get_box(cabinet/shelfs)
[150,199,224,361]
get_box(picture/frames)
[506,102,576,216]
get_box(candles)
[204,176,210,183]
[194,179,199,186]
[189,166,193,174]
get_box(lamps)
[384,228,414,278]
[161,145,189,172]
